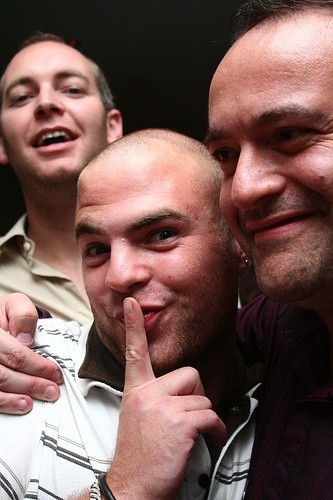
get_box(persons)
[0,126,255,498]
[0,0,333,499]
[0,30,128,322]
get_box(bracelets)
[88,482,103,499]
[97,471,117,499]
